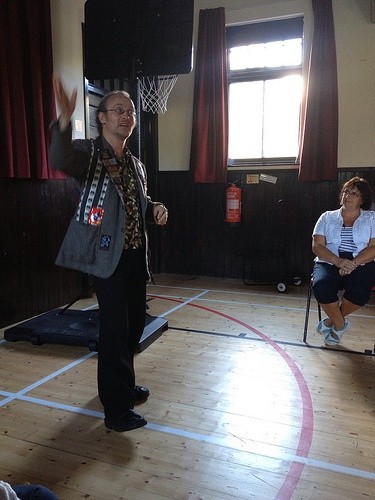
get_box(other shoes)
[104,409,147,432]
[324,318,352,345]
[134,386,149,400]
[316,317,334,335]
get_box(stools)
[303,272,375,342]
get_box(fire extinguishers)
[224,178,242,223]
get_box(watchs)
[352,259,356,264]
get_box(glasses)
[101,108,136,117]
[342,191,358,198]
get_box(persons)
[311,177,375,346]
[48,79,168,433]
